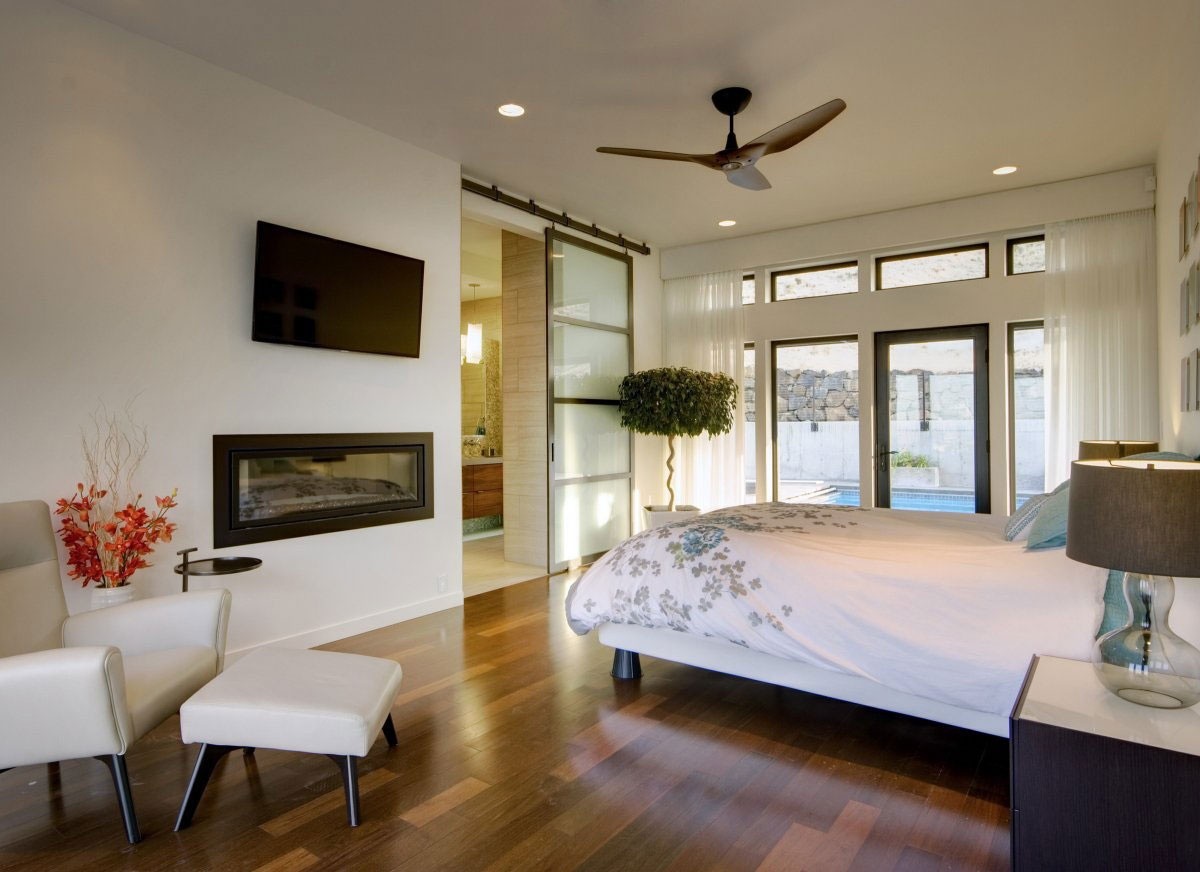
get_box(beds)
[566,500,1200,738]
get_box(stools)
[172,645,403,831]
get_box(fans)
[596,87,847,192]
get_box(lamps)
[465,282,484,365]
[1066,439,1200,708]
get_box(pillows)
[1002,451,1193,550]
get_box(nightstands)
[1007,652,1200,872]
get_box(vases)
[90,581,136,611]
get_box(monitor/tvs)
[251,220,424,358]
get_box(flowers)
[52,392,180,589]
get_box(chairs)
[0,497,232,844]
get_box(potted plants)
[617,365,743,528]
[888,452,939,488]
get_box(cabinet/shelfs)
[463,462,504,525]
